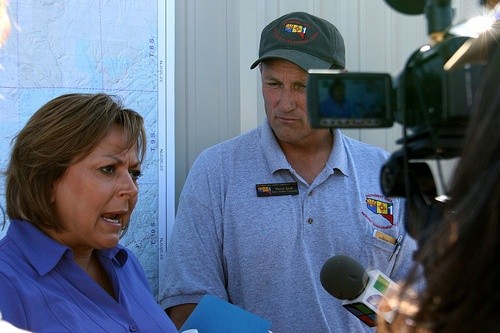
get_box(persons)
[159,11,428,333]
[319,77,383,117]
[0,92,179,333]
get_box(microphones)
[319,255,401,327]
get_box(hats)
[250,12,345,71]
